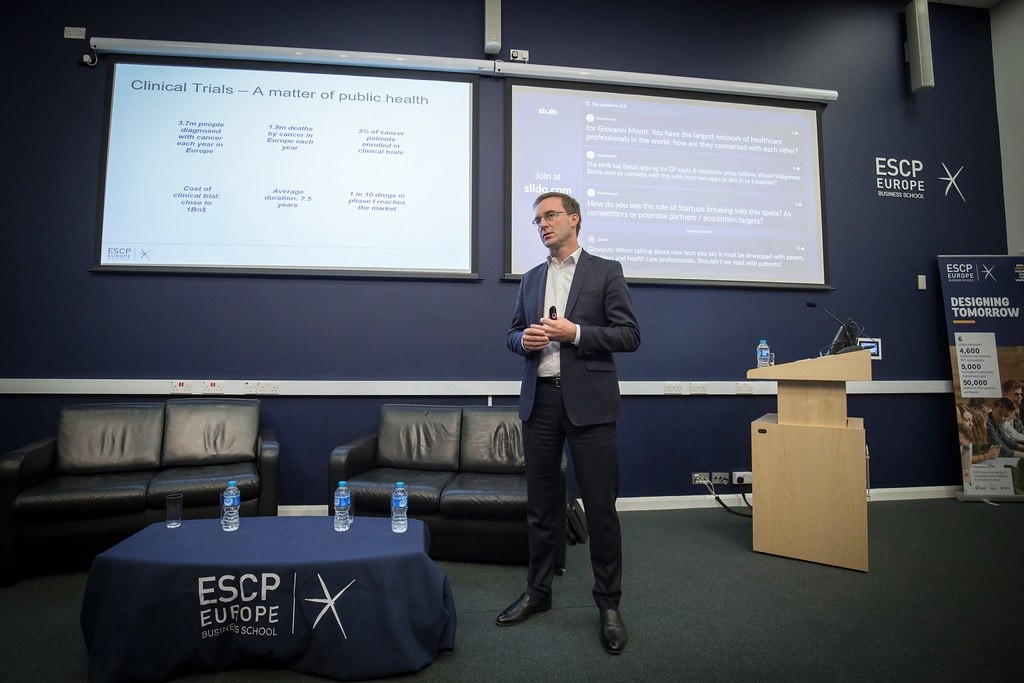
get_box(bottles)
[756,340,770,368]
[390,481,409,535]
[333,480,351,532]
[221,480,240,533]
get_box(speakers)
[905,0,935,93]
[484,0,501,54]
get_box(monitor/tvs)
[827,317,853,355]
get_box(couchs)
[329,403,576,575]
[0,399,279,587]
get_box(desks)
[79,517,457,683]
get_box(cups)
[165,493,183,529]
[219,492,226,523]
[770,353,775,366]
[348,492,355,524]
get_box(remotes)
[549,306,557,320]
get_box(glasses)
[1014,393,1024,396]
[532,211,574,224]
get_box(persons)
[1002,380,1023,433]
[971,405,988,456]
[495,191,641,655]
[956,403,999,464]
[986,398,1024,458]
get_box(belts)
[537,377,561,387]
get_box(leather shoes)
[496,592,551,626]
[600,608,628,654]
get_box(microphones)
[806,302,863,354]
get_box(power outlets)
[692,473,709,484]
[733,471,752,484]
[711,472,729,485]
